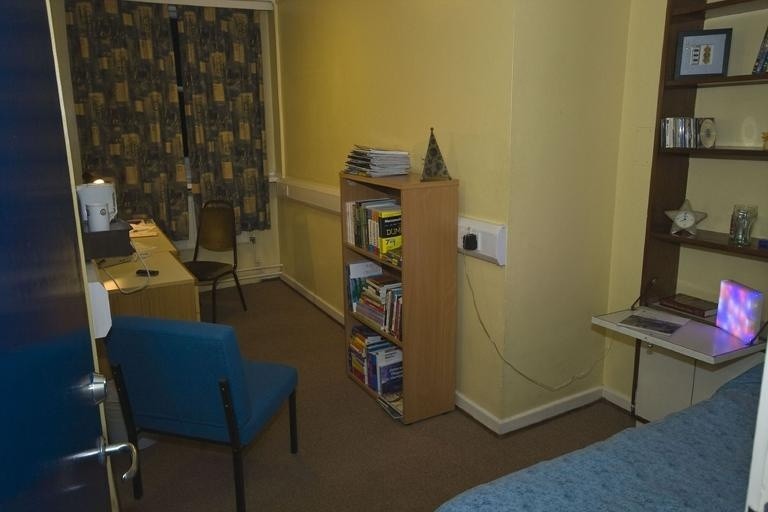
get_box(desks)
[88,217,202,321]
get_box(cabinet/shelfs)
[590,0,768,430]
[336,168,460,425]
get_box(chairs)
[100,318,301,512]
[183,198,248,324]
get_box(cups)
[728,203,758,246]
[86,202,118,232]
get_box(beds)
[429,363,764,511]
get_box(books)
[616,307,692,337]
[344,196,403,396]
[659,293,719,318]
[344,144,411,179]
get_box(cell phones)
[136,270,159,276]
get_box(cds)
[700,119,716,149]
